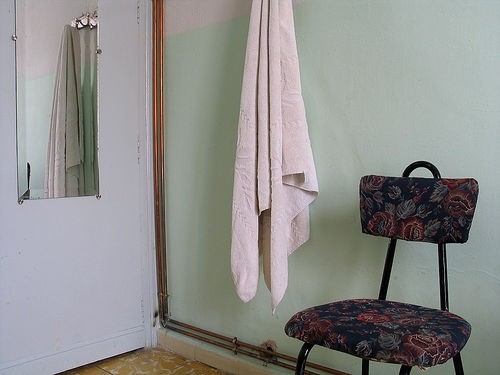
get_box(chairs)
[283,161,480,375]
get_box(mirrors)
[12,0,102,205]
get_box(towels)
[230,0,319,315]
[44,24,84,198]
